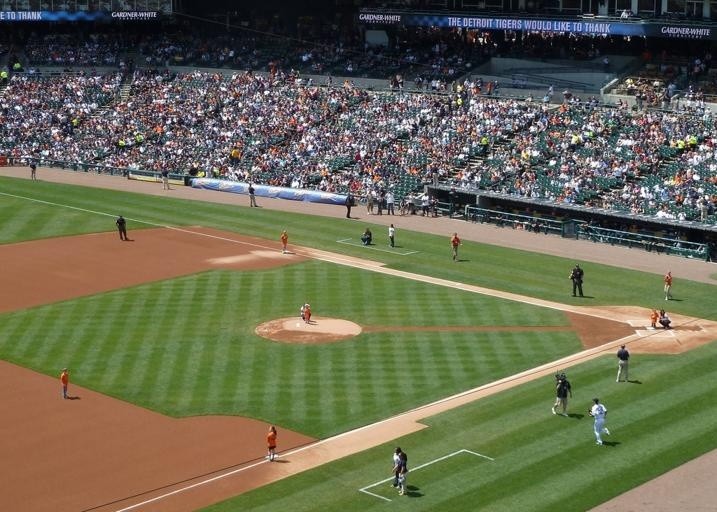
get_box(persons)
[616,344,629,382]
[305,304,310,324]
[569,264,584,297]
[651,310,659,327]
[266,426,277,462]
[61,368,69,399]
[589,398,610,446]
[390,447,402,489]
[392,452,408,496]
[552,373,572,417]
[664,272,672,300]
[659,310,671,329]
[301,306,311,321]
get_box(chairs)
[0,70,716,255]
